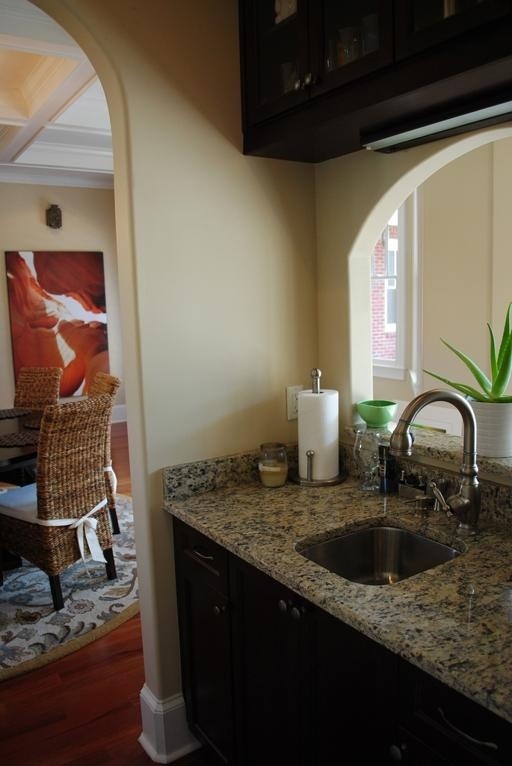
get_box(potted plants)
[421,302,512,458]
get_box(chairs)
[0,366,120,610]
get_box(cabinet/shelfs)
[237,0,512,130]
[171,509,512,765]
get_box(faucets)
[390,389,479,534]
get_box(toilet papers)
[298,390,341,483]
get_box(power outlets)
[286,385,305,422]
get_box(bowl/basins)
[356,397,399,429]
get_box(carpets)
[0,480,141,680]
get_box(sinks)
[295,514,469,589]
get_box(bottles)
[258,440,288,487]
